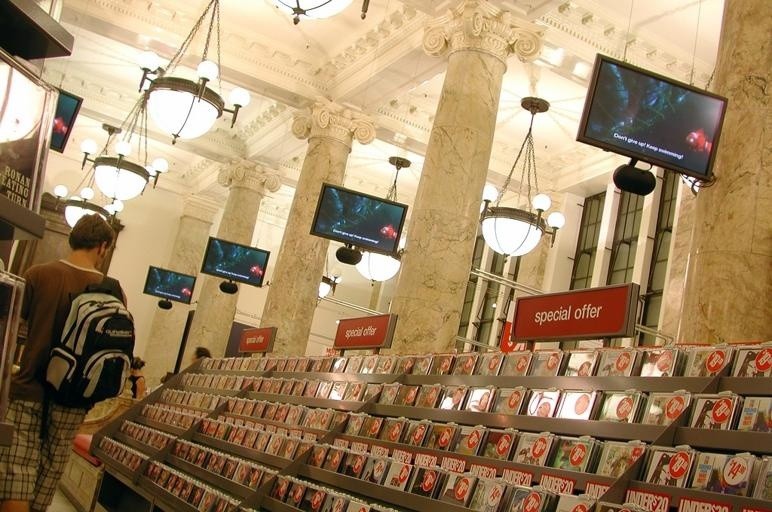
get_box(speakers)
[335,247,362,265]
[219,282,238,294]
[613,165,656,196]
[158,300,173,309]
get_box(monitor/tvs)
[199,236,271,288]
[576,52,728,181]
[309,182,409,256]
[50,86,84,153]
[143,265,196,305]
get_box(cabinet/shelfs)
[88,340,772,512]
[5,191,122,375]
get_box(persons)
[128,357,145,400]
[0,212,127,511]
[192,347,210,364]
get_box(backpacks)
[33,276,135,409]
